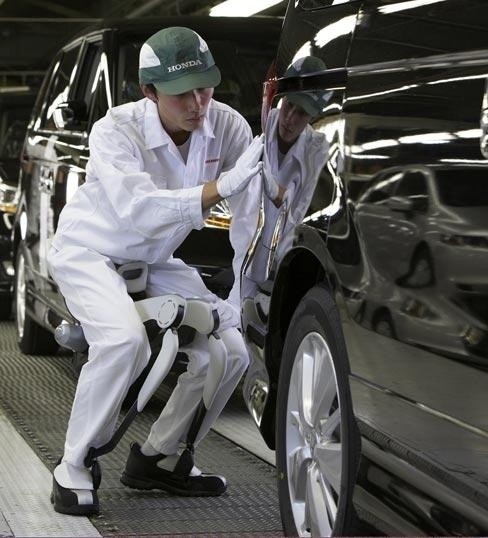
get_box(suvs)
[259,4,487,535]
[8,1,287,377]
[0,70,52,328]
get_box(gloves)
[216,134,268,201]
[262,139,279,203]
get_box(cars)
[351,157,486,296]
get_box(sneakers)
[48,457,101,520]
[117,440,227,500]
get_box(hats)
[279,55,336,116]
[136,25,223,97]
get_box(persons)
[42,26,266,516]
[239,54,336,321]
